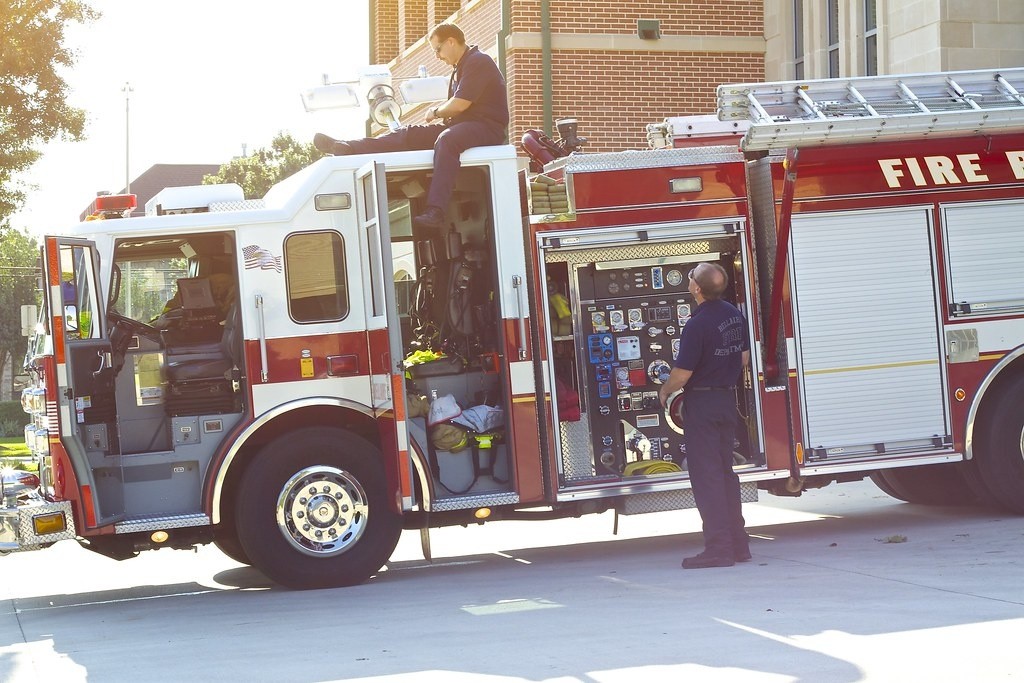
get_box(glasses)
[688,269,700,288]
[434,37,449,52]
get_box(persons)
[314,25,508,227]
[660,264,751,568]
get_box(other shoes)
[314,133,353,156]
[682,542,752,569]
[414,207,444,228]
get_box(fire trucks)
[0,70,1023,588]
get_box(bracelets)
[433,107,439,118]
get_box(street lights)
[119,80,136,193]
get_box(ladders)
[716,66,1023,151]
[645,114,754,150]
